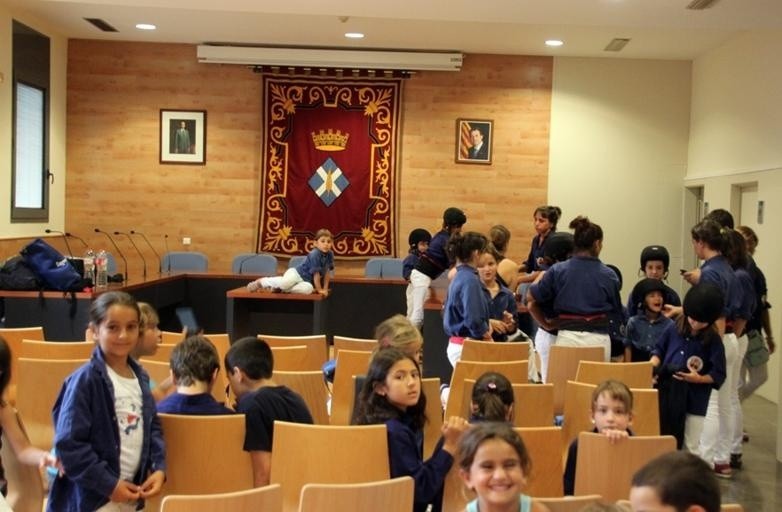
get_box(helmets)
[633,278,665,306]
[409,228,431,244]
[444,207,466,227]
[682,284,724,323]
[640,245,669,271]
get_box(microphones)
[45,229,78,273]
[65,233,88,248]
[131,231,161,272]
[94,228,127,279]
[114,232,146,277]
[165,234,170,273]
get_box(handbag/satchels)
[744,330,769,368]
[0,238,83,291]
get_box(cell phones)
[680,269,686,273]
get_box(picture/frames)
[454,117,494,166]
[156,108,208,166]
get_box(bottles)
[96,250,108,287]
[84,248,96,285]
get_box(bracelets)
[766,337,773,340]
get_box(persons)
[155,334,234,413]
[477,224,538,381]
[129,302,176,402]
[247,229,334,298]
[44,291,167,510]
[375,314,424,366]
[0,340,66,510]
[663,208,774,479]
[456,422,545,510]
[629,451,721,509]
[648,283,727,450]
[622,279,673,363]
[467,126,489,162]
[353,347,469,510]
[627,246,682,317]
[512,206,561,303]
[176,120,192,155]
[526,215,629,385]
[224,336,314,489]
[410,207,467,331]
[562,380,634,495]
[468,371,514,426]
[439,230,495,413]
[403,228,432,318]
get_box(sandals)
[713,453,743,478]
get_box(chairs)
[1,252,746,511]
